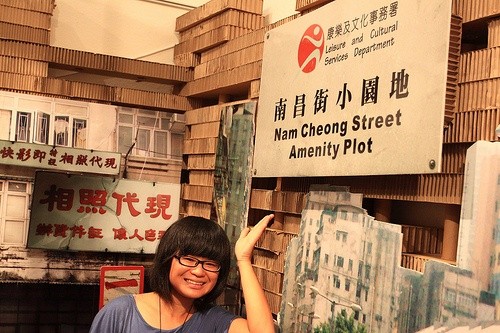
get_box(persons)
[90,214,275,333]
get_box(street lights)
[309,286,360,332]
[288,303,320,333]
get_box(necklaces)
[158,295,193,333]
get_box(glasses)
[172,254,222,272]
